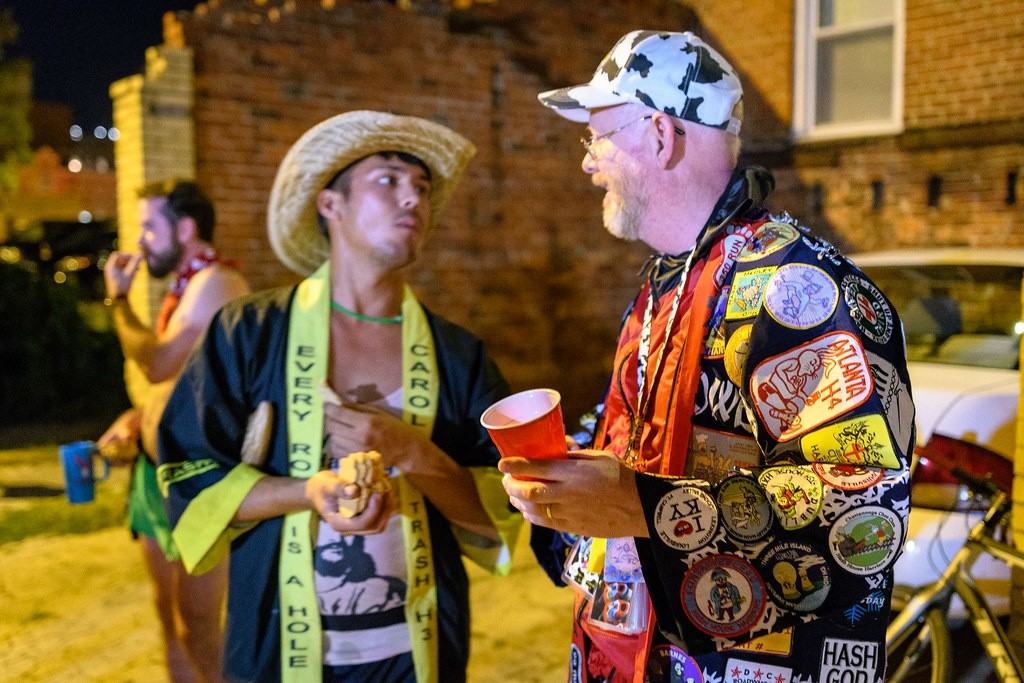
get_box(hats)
[537,29,745,135]
[265,107,479,278]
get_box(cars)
[842,251,1024,619]
[0,214,119,303]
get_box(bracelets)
[104,293,129,310]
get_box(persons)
[97,176,250,683]
[495,29,915,683]
[154,111,523,683]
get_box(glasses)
[580,115,684,161]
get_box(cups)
[61,441,109,504]
[480,389,568,482]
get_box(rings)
[546,503,553,519]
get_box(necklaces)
[326,298,404,329]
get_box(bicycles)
[886,461,1024,683]
